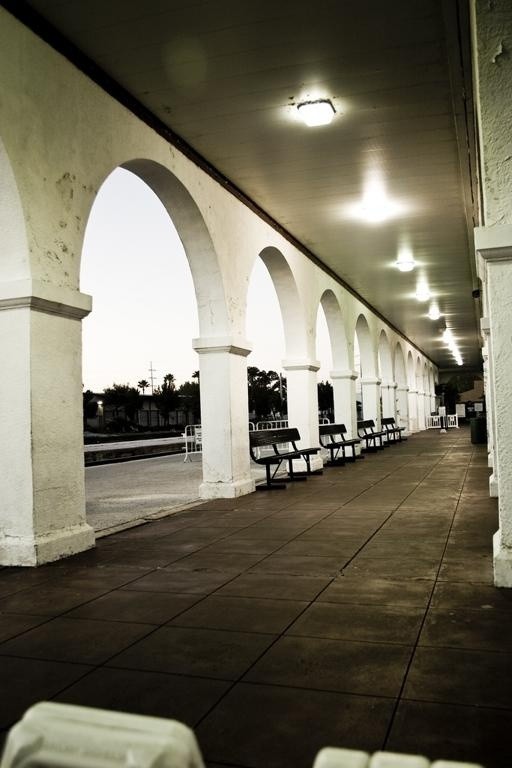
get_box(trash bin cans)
[470,418,487,444]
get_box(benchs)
[249,428,323,490]
[381,418,407,444]
[357,420,386,453]
[319,424,364,467]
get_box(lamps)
[297,99,336,128]
[396,261,414,272]
